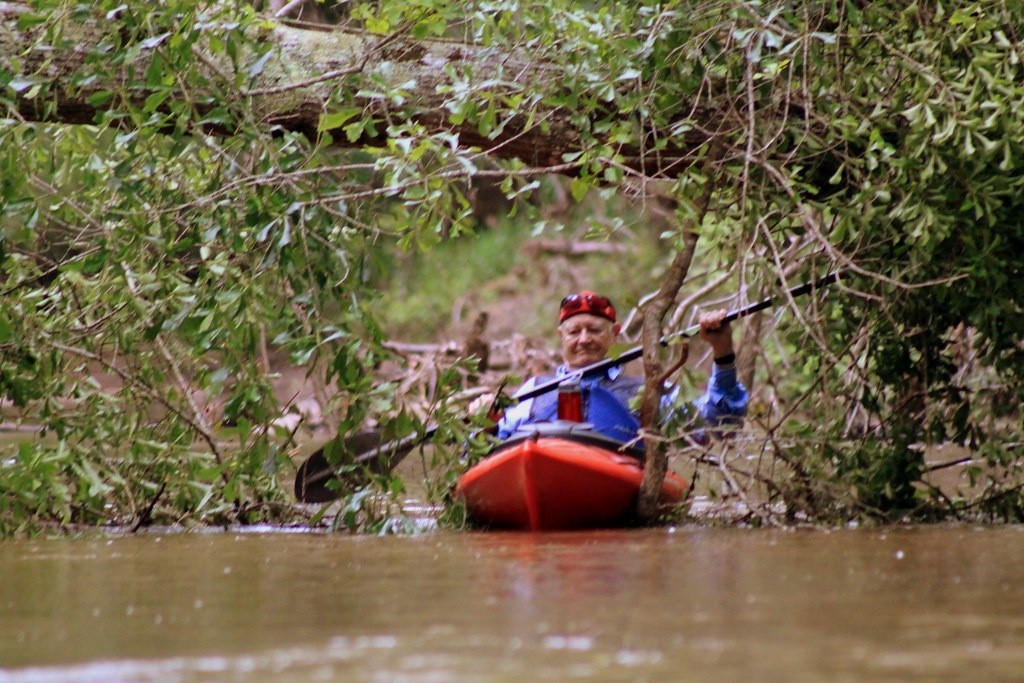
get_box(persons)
[465,291,750,460]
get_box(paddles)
[294,265,865,505]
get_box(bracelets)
[713,351,737,366]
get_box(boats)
[453,417,693,533]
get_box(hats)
[559,291,616,324]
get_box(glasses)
[561,293,613,312]
